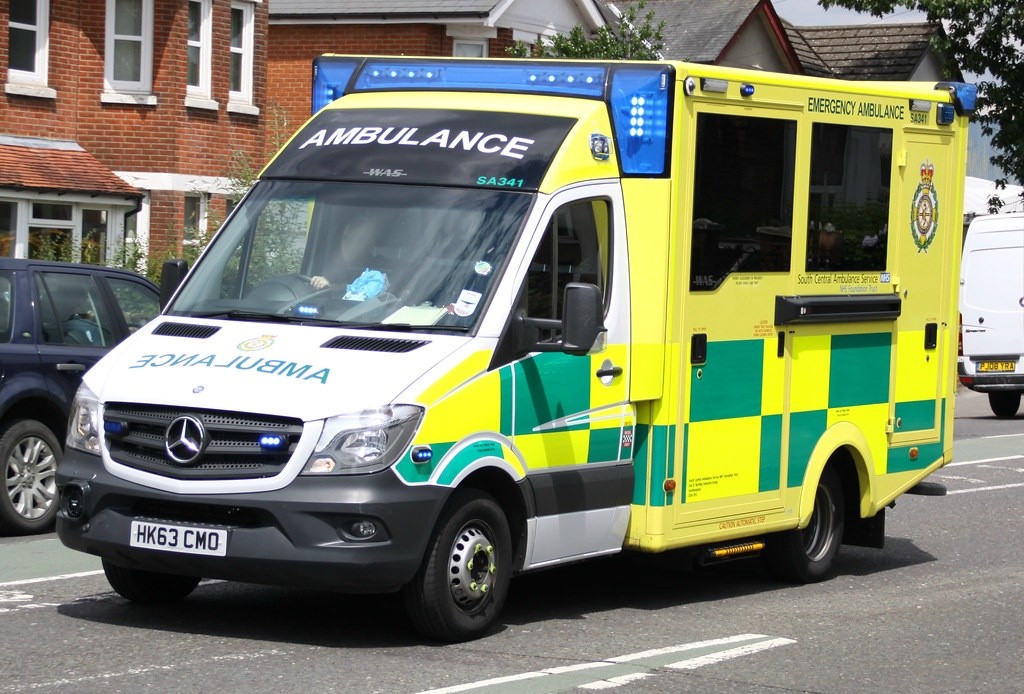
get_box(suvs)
[1,252,176,538]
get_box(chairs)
[42,294,74,344]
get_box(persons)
[309,213,389,292]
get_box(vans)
[956,210,1024,418]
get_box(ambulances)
[56,48,978,643]
